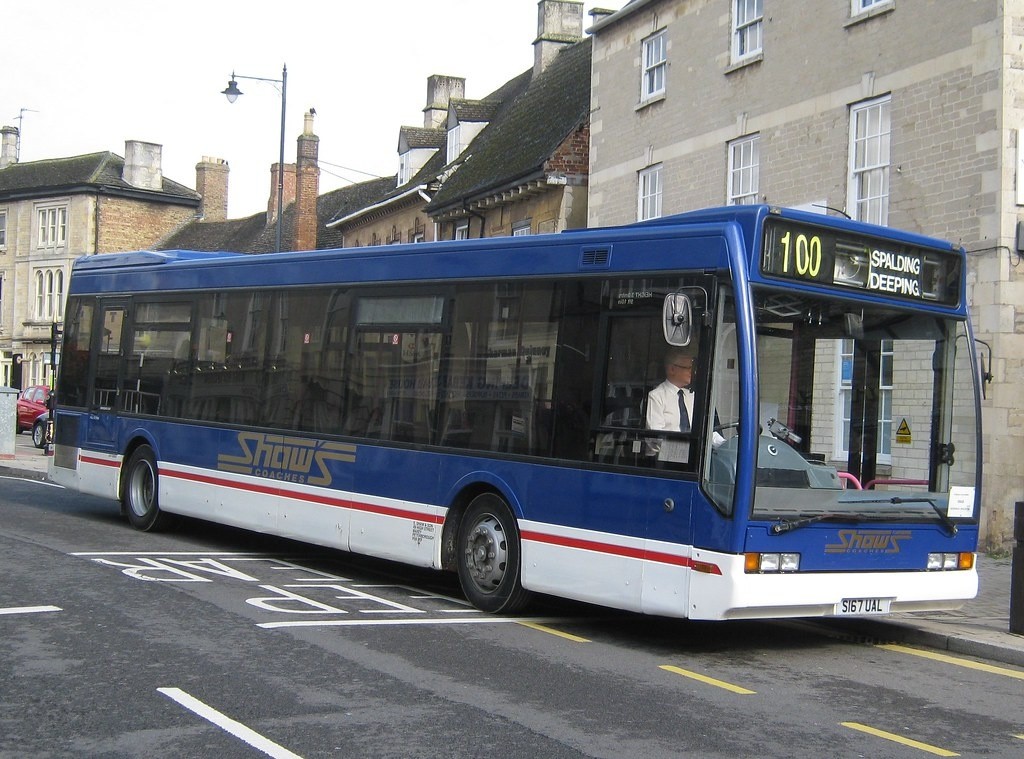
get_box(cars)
[16,385,50,434]
[32,409,56,449]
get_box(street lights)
[216,63,288,254]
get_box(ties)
[677,390,691,432]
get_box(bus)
[54,203,994,624]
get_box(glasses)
[672,363,693,371]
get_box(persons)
[640,352,727,463]
